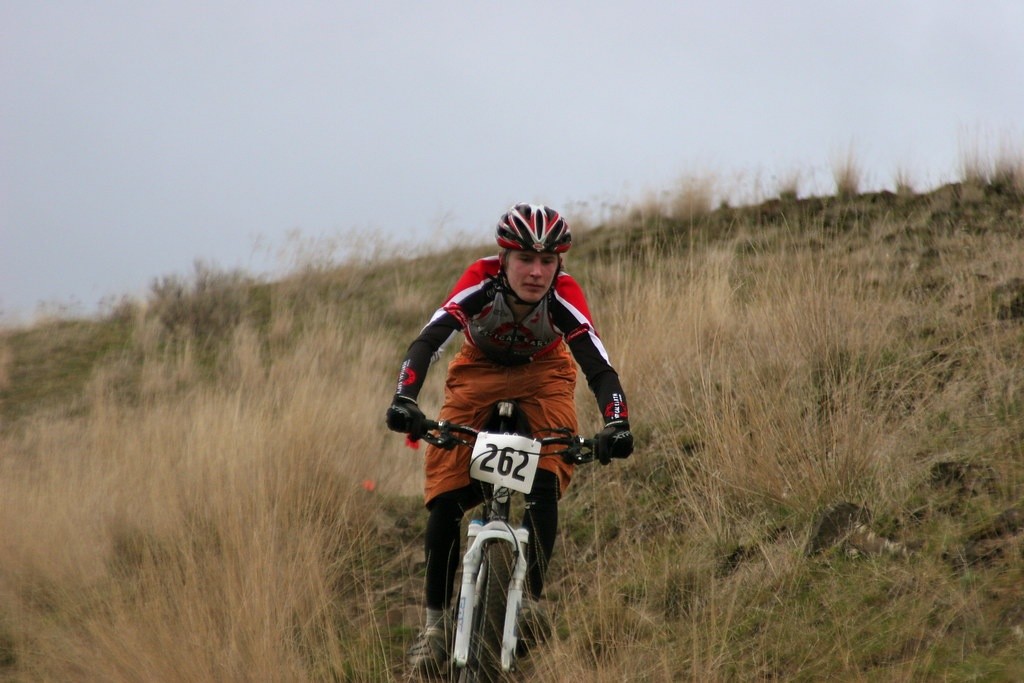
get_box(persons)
[386,201,633,674]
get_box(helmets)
[495,202,571,254]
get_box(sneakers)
[407,625,454,673]
[520,590,552,643]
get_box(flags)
[405,433,419,450]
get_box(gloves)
[386,395,428,442]
[593,420,634,466]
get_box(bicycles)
[404,400,599,683]
